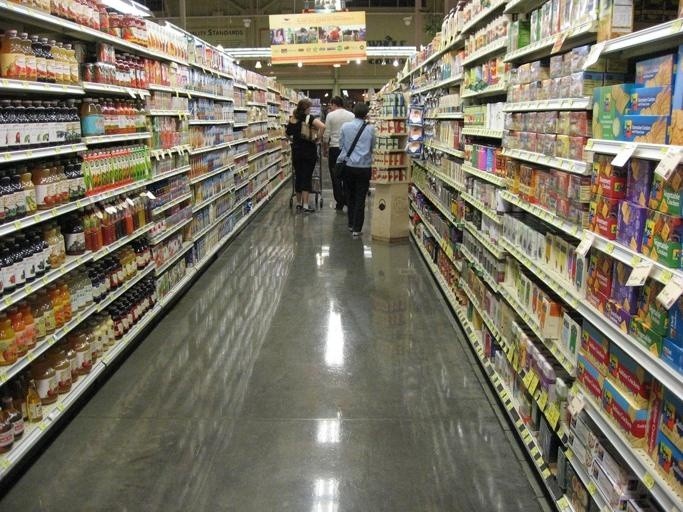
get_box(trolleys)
[283,142,325,210]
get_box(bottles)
[0,1,160,455]
[152,23,193,239]
[459,1,509,186]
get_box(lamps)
[221,16,416,69]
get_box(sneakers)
[346,226,353,232]
[353,231,365,237]
[303,206,316,212]
[334,202,344,210]
[296,203,303,210]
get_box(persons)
[336,102,377,236]
[271,25,366,45]
[324,95,355,211]
[286,98,326,214]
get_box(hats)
[353,103,369,116]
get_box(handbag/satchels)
[334,161,347,182]
[300,114,318,142]
